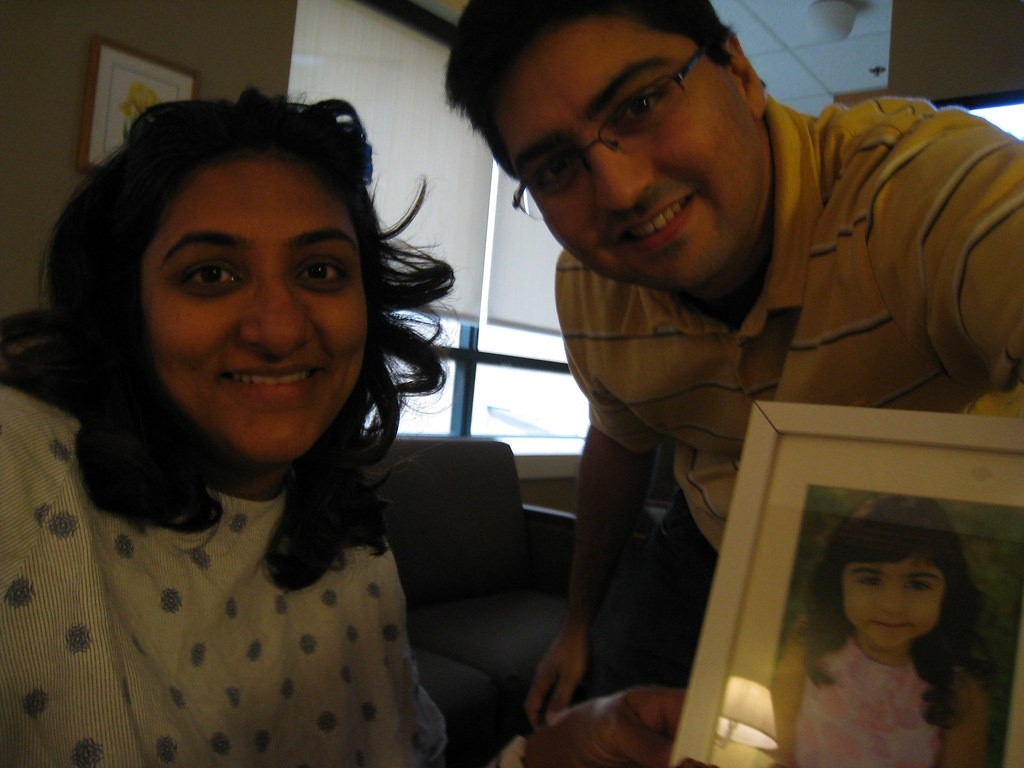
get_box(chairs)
[364,440,577,768]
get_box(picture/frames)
[76,34,202,174]
[669,401,1024,768]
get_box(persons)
[759,492,998,768]
[445,0,1024,727]
[0,86,690,768]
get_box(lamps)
[807,0,856,45]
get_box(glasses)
[511,45,707,222]
